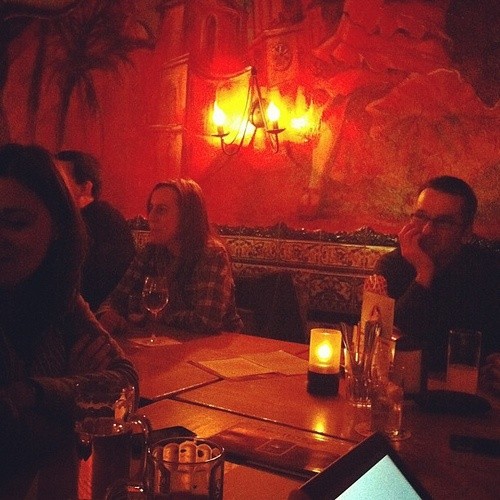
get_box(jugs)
[72,416,150,500]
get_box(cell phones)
[405,389,490,414]
[132,426,197,455]
[448,434,500,457]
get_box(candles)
[266,103,284,154]
[202,102,232,156]
[308,328,342,397]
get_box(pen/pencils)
[337,320,383,407]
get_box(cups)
[102,437,224,500]
[369,364,402,435]
[446,330,481,396]
[344,345,390,407]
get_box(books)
[197,420,354,480]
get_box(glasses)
[410,212,453,231]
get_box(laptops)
[299,428,433,500]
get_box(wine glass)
[141,276,169,344]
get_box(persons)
[92,178,245,335]
[44,151,137,317]
[372,175,500,368]
[0,143,140,500]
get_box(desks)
[97,327,500,500]
